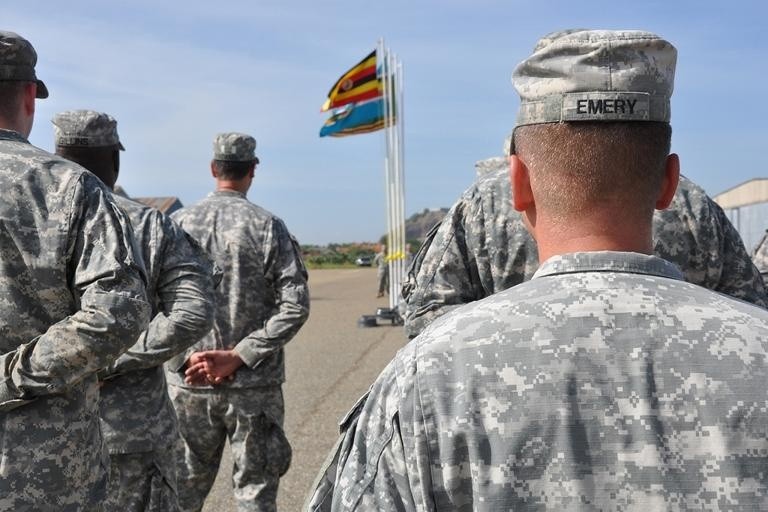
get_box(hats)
[0,30,48,98]
[510,28,677,154]
[52,110,125,151]
[214,133,259,164]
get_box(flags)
[319,49,377,110]
[319,63,396,138]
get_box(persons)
[0,30,151,512]
[48,108,215,512]
[405,27,766,340]
[165,130,310,512]
[304,32,767,512]
[372,241,416,297]
[748,233,766,283]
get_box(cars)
[354,257,371,266]
